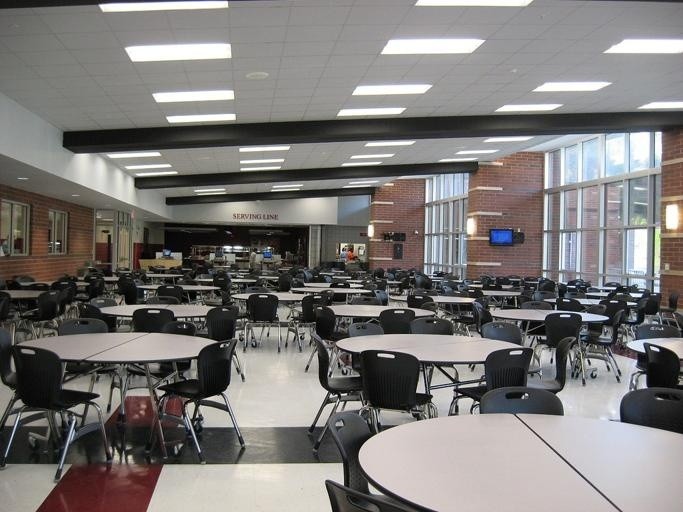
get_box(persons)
[363,246,368,255]
[347,248,357,261]
[358,246,365,257]
[340,248,347,262]
[250,247,257,269]
[175,247,223,279]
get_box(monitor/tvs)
[263,252,272,258]
[393,232,406,241]
[162,249,171,256]
[489,229,513,246]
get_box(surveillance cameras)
[415,231,419,235]
